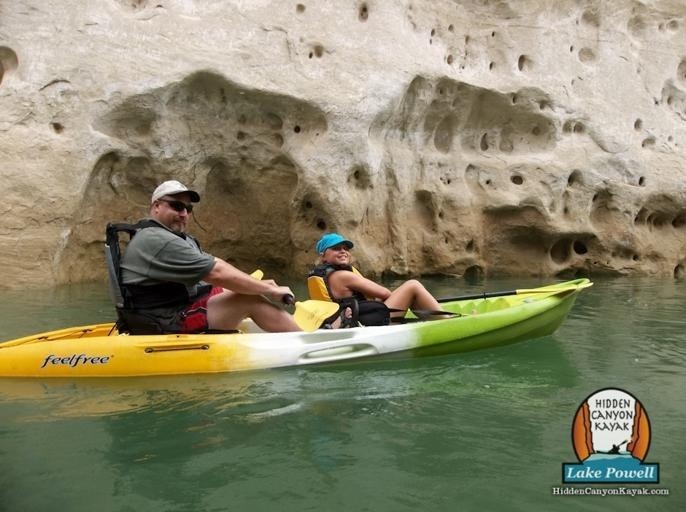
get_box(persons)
[113,180,353,334]
[312,233,476,326]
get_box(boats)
[0,277,590,377]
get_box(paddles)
[437,283,594,303]
[247,269,340,332]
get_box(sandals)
[324,309,352,329]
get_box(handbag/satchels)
[352,301,390,326]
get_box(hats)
[151,180,200,206]
[316,233,354,256]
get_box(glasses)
[159,198,194,213]
